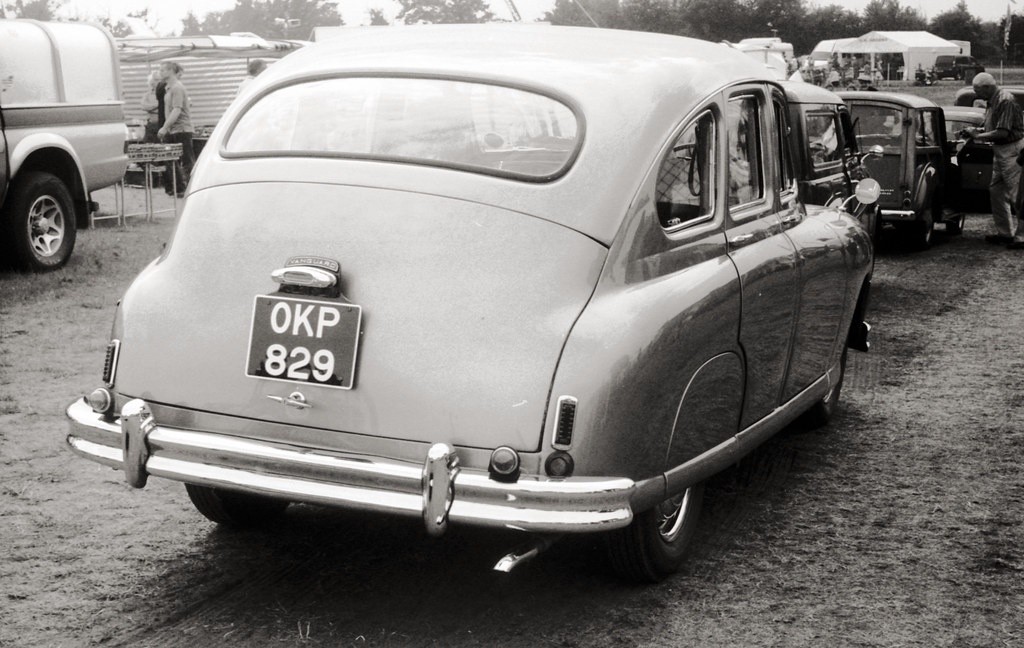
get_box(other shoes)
[989,234,1021,253]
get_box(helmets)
[972,72,996,86]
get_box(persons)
[784,54,884,90]
[136,61,194,197]
[956,73,1023,250]
[237,60,266,91]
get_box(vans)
[806,38,856,66]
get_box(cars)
[63,23,883,582]
[0,17,129,273]
[934,56,985,81]
[776,76,1024,260]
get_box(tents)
[808,31,962,82]
